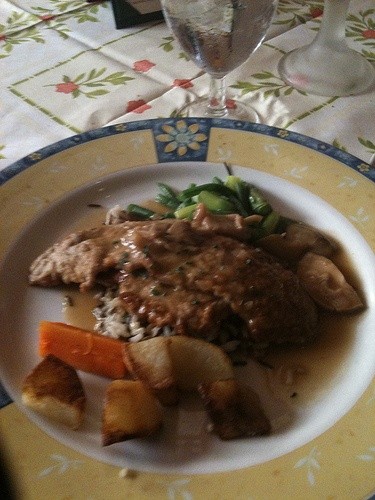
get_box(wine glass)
[160,0,279,126]
[277,0,375,97]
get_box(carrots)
[39,320,130,381]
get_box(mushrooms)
[297,253,365,315]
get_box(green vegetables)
[127,174,292,242]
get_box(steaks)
[28,204,319,361]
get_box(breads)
[22,354,274,446]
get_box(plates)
[1,116,375,500]
